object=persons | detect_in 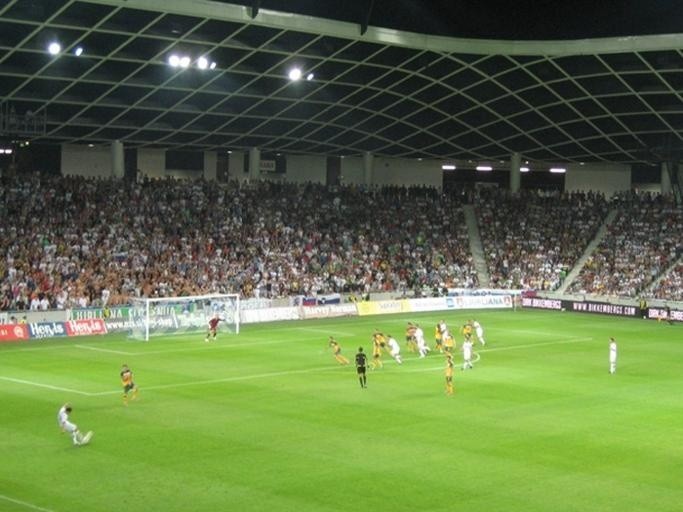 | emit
[461,319,485,369]
[435,320,454,395]
[355,329,402,388]
[473,184,683,299]
[610,337,618,374]
[406,321,431,358]
[639,296,671,323]
[1,166,479,312]
[120,363,139,402]
[329,336,350,364]
[57,402,84,446]
[206,314,226,341]
[9,316,27,325]
[102,304,112,318]
